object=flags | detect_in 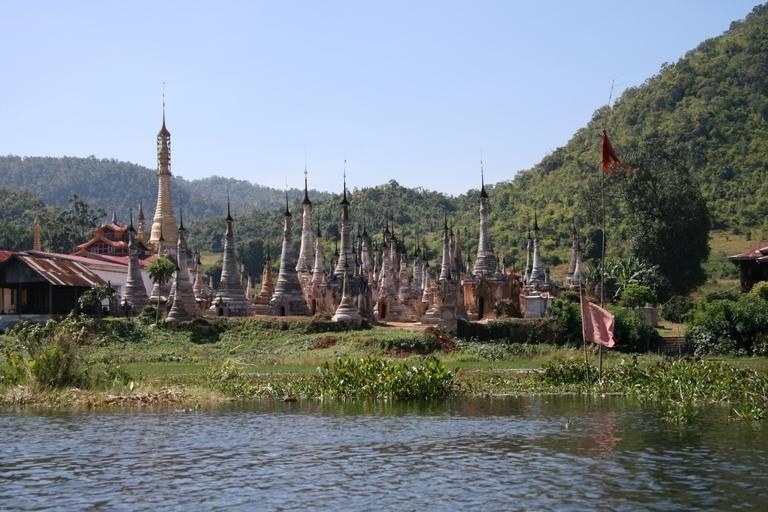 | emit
[579,294,616,347]
[600,129,640,177]
